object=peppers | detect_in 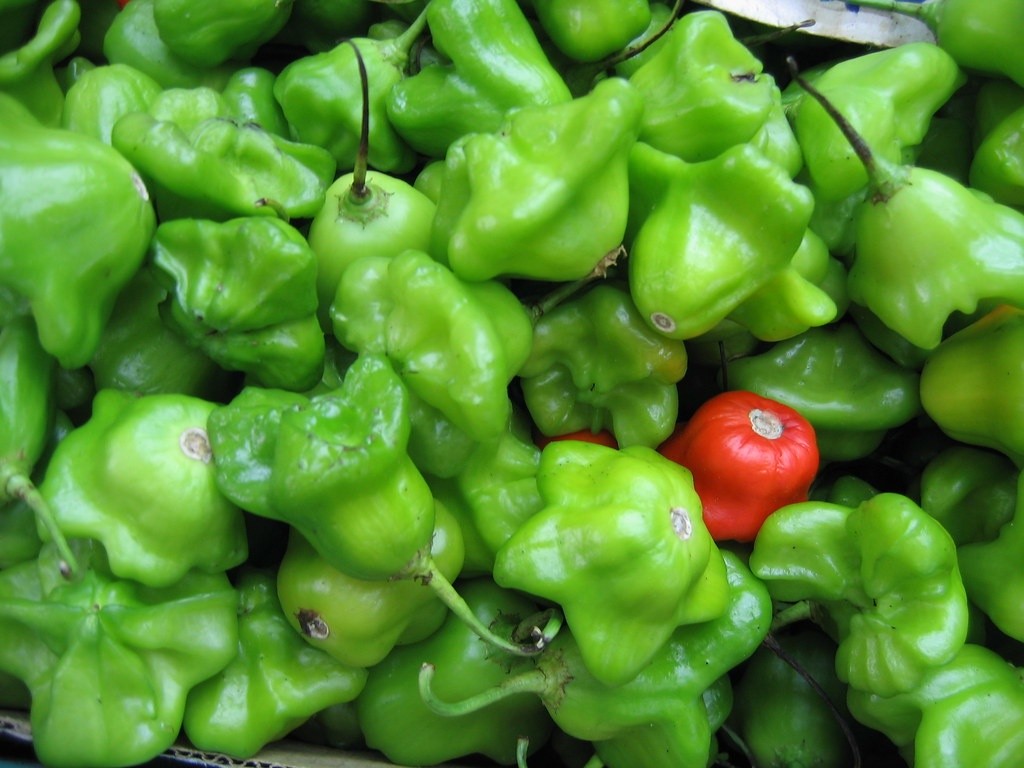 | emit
[0,0,1024,768]
